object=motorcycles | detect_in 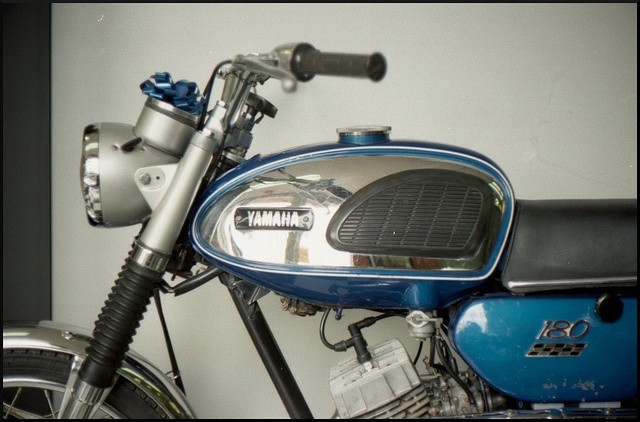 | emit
[0,41,637,421]
